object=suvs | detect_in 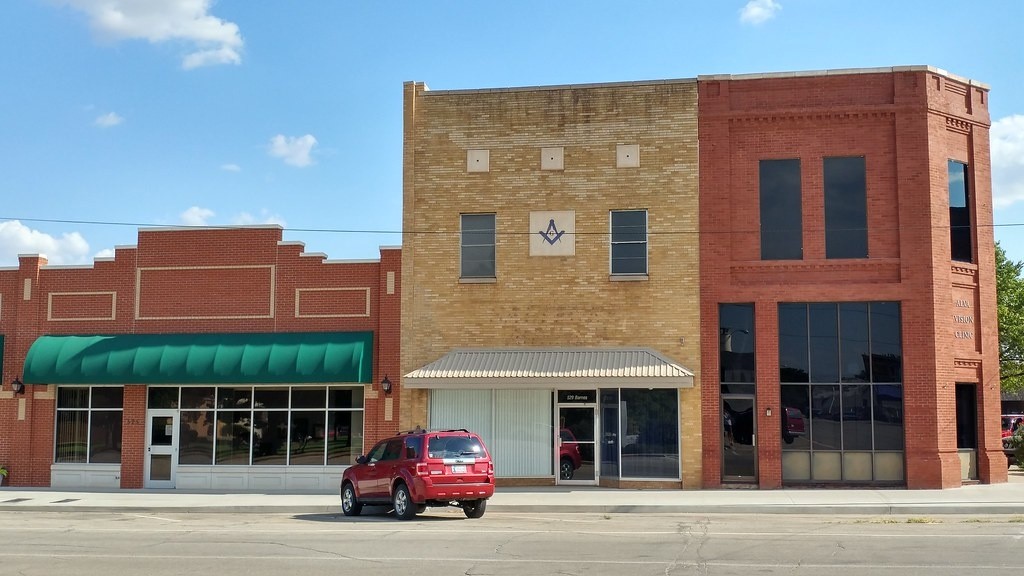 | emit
[560,429,582,480]
[340,429,496,520]
[781,406,806,436]
[1002,415,1024,468]
